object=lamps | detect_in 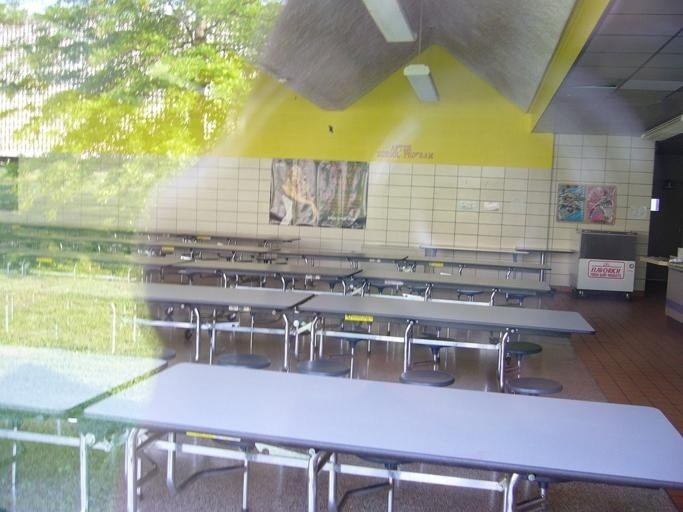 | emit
[361,1,441,105]
